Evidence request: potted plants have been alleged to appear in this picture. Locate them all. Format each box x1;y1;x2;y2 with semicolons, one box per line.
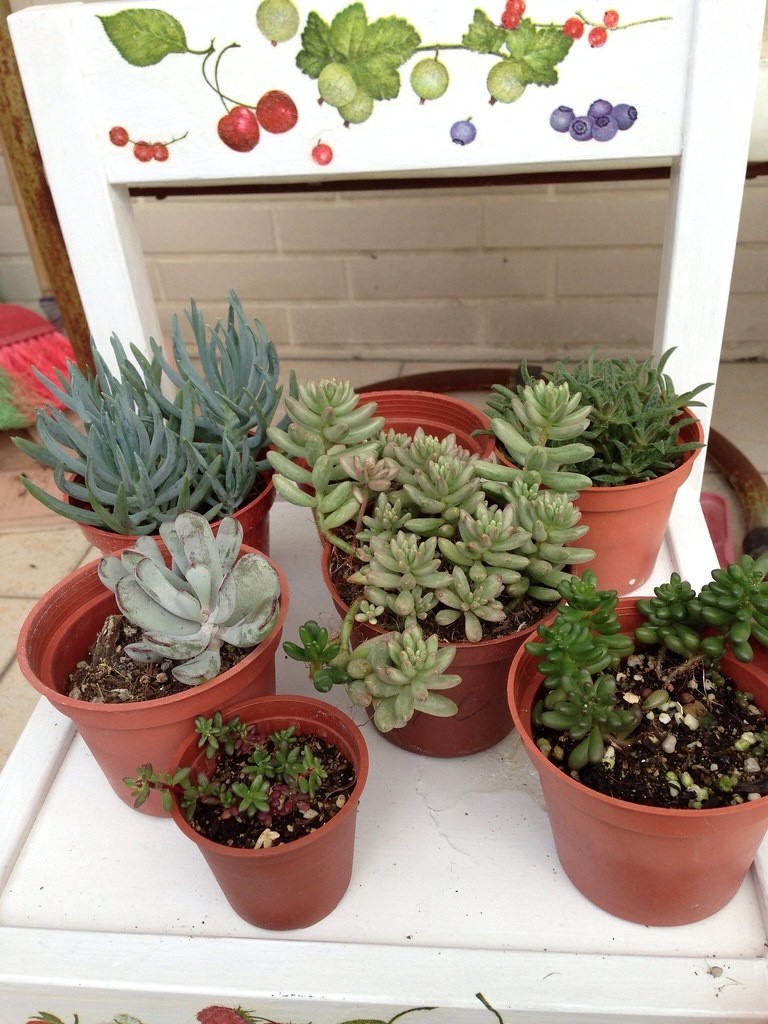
11;286;275;558
16;509;290;817
262;374;495;550
283;378;596;757
468;342;714;599
507;545;768;927
119;695;368;931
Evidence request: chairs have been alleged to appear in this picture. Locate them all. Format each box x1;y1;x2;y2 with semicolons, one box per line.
0;3;768;1024
